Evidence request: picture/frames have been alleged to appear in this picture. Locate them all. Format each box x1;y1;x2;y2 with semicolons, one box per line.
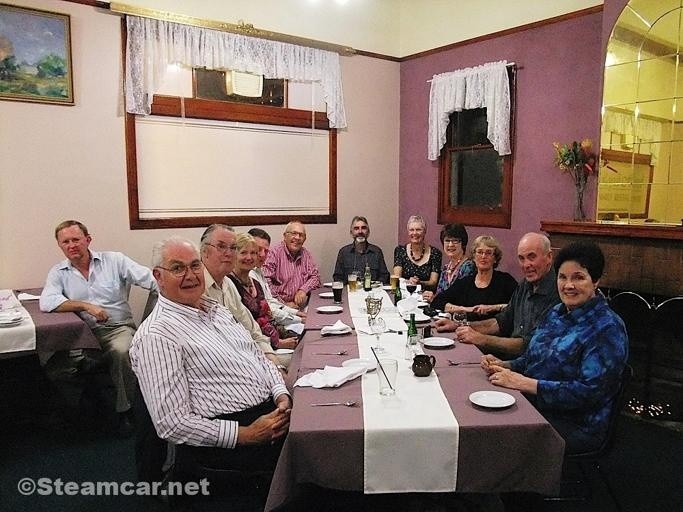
0;2;75;106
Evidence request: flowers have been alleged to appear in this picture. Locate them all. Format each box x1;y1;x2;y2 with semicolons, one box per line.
551;135;609;177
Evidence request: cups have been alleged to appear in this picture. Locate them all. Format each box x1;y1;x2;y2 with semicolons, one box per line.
332;271;467;397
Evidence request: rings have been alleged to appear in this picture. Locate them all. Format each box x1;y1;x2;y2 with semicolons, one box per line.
496;376;497;379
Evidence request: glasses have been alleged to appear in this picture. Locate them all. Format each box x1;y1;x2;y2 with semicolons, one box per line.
475;250;493;256
156;262;202;277
205;243;238;253
287;232;306;239
444;239;458;244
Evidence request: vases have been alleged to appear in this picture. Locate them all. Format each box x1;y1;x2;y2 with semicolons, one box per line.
572;175;588;222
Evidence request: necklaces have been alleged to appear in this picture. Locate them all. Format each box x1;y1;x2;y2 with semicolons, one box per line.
447;258;463;274
410;244;426;262
233;271;253;295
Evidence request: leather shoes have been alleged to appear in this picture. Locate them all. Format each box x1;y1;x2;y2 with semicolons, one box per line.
116;409;135;440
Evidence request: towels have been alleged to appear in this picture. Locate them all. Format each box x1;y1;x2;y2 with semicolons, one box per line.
396;295;423;316
291;362;377;389
320;319;352;339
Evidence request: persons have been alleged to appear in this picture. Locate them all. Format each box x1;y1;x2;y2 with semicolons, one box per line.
198;223;292;390
332;216;391;285
126;236;292;469
38;220;162;439
422;221;476;304
430;234;519;321
481;240;630;512
394;214;442;293
262;220;322;312
435;232;563;359
246;228;307;334
227;233;302;349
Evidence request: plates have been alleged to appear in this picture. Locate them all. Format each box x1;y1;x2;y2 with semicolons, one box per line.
317;282;516;408
0;310;24;327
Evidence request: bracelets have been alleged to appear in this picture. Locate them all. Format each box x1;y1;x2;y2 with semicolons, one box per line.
500;304;502;312
277;365;286;369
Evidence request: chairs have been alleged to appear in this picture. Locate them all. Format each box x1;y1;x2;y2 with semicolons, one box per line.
121;357;253;512
569;365;636;512
126;283;158;328
